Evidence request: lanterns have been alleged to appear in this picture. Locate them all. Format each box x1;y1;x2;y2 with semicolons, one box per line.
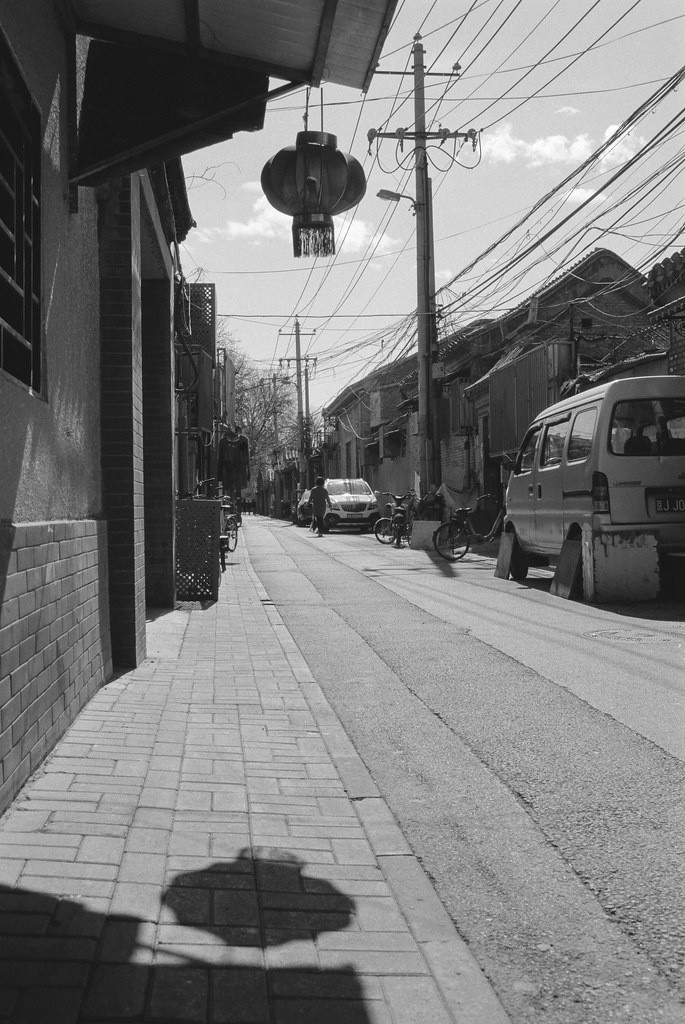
259;131;365;257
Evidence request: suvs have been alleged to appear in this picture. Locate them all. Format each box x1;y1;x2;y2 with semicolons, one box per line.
320;478;381;529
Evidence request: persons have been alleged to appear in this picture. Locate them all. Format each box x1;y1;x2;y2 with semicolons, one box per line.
240;498;256;515
306;477;332;536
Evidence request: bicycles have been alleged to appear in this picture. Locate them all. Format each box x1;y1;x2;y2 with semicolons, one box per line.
431;494;507;562
189;477;239;552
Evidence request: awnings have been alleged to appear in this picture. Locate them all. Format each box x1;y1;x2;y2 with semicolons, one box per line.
52;1;399;95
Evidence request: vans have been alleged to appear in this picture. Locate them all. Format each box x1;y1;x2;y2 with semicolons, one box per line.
503;375;685;582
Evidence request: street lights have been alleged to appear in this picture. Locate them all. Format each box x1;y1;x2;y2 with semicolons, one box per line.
376;186;428;497
281;380;306;494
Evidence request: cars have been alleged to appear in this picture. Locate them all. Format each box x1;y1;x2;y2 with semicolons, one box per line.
373;491;444;548
296;489;316;527
